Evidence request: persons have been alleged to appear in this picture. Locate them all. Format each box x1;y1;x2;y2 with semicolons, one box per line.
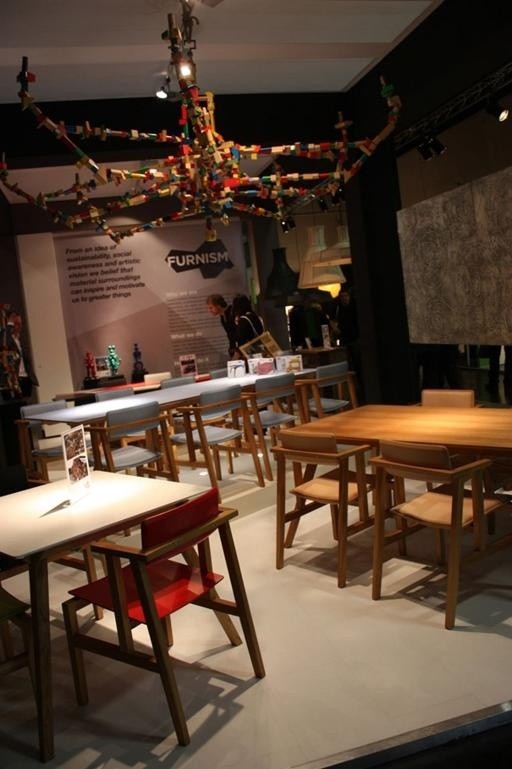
6;313;41;405
206;288;354;436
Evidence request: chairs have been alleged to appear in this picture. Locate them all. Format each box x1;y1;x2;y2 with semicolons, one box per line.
293;360;359;421
209;365;266;431
159;375;225;433
410;388;482;412
61;485;267;746
224;372;312;479
169;384;269;503
87;406;180;484
23;400;84;476
265;427;397;598
92;373;129;386
142;370;173;383
367;435;505;636
90;383;183;463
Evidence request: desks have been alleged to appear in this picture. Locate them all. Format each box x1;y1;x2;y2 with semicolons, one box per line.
21;361;351;478
277;402;510;563
42;361;242;395
0;465;208;750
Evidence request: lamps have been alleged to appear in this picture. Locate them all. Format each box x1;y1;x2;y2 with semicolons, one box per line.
2;2;409;261
